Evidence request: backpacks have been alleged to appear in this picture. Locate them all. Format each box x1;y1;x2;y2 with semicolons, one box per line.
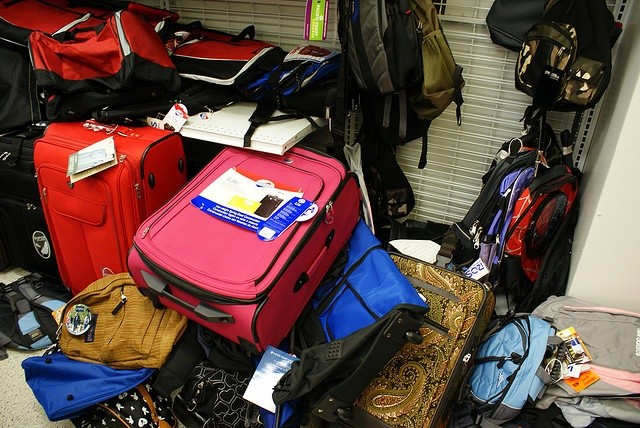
0;268;73;360
450;123;586;313
461;295;640;426
373;218;450;256
487;1;623;125
338;0;465;148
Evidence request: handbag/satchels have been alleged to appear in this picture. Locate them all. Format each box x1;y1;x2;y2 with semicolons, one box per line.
327;124;414;226
0;0;339;126
266;214;428;428
22;271;261;426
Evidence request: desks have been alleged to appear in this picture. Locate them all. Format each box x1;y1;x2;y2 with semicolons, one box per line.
138;99;330;169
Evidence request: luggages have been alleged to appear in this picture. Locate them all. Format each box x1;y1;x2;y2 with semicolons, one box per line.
33;120;186;298
0;125;49;269
124;143;363;364
338;249;495;427
